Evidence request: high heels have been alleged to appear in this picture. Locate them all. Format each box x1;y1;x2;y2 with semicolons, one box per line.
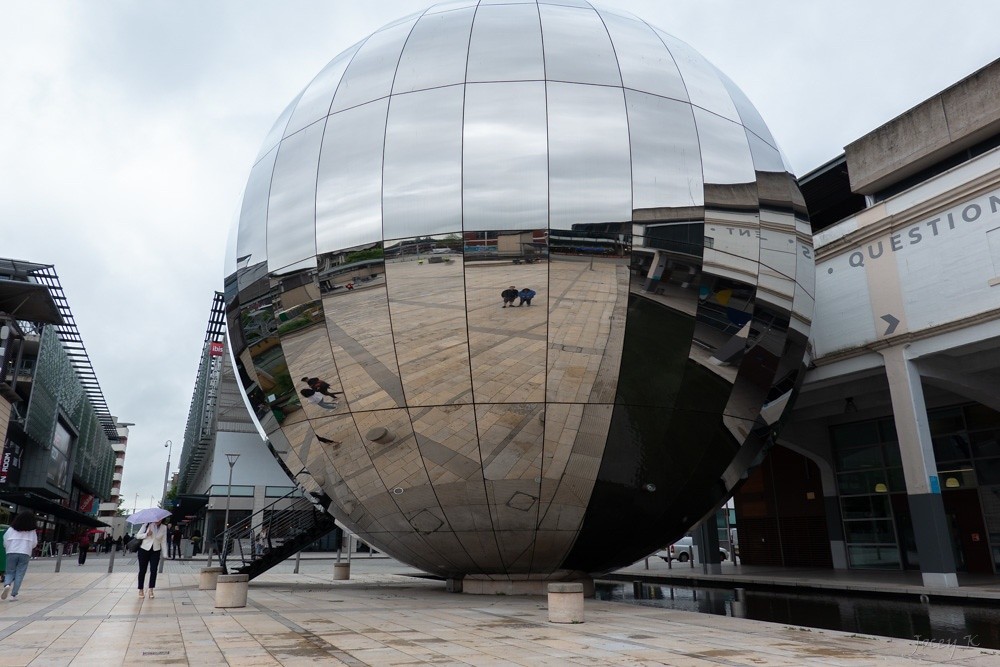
147;588;155;598
139;590;145;599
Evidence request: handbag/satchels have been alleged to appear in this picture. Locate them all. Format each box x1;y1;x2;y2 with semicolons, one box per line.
127;538;143;552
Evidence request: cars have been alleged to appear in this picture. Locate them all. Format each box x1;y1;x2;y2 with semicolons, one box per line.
656;537;730;563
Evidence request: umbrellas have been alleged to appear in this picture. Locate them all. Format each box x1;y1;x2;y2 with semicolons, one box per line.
89;529;104;533
126;508;172;525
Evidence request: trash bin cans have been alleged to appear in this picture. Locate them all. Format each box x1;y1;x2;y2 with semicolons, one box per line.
0;529;6;582
184;541;194;558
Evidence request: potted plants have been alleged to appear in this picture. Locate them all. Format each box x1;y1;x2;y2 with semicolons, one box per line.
192;536;200;556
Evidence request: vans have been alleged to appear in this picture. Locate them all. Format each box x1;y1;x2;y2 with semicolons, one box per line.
729;527;740;556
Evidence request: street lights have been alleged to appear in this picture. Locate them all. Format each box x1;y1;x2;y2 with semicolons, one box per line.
220;453;241;567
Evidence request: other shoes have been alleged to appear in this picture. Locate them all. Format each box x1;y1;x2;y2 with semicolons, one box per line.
517;304;523;308
526;305;531;308
1;586;10;600
331;397;341;400
502;305;515;308
335;401;339;409
333;440;342;446
10;595;19;602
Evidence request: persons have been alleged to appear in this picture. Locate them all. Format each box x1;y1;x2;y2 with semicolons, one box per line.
2;511;38;601
501;285;518;309
300;389;339;412
316;434;342;446
257;527;267;559
77;523;201;567
136;518;167;599
518;288;536;307
301;377;343;401
